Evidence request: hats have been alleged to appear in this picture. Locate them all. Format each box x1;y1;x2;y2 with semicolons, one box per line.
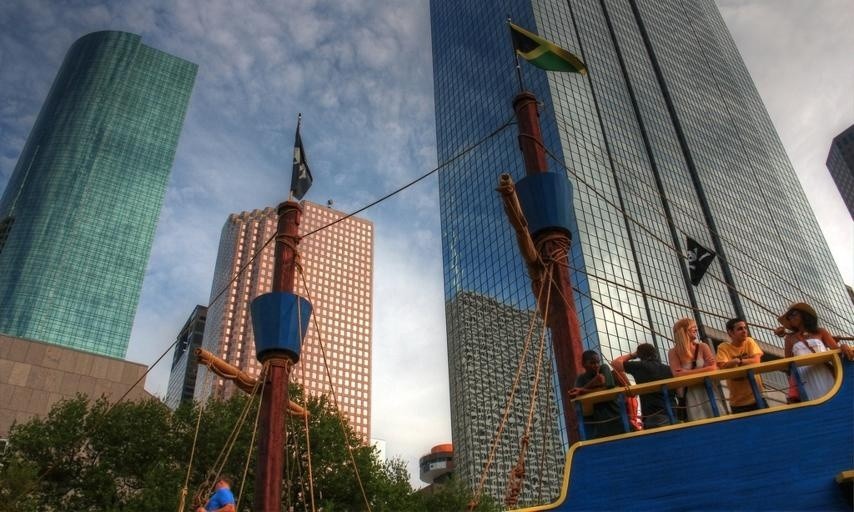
777;303;817;331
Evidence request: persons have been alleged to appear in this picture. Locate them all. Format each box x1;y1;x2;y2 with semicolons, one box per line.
567;303;854;438
195;474;237;512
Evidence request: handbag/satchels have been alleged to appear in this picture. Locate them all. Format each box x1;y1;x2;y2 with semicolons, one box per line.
677;398;688;420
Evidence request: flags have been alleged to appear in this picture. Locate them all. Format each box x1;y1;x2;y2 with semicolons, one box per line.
509;23;589;78
687;235;717;286
290;121;314;201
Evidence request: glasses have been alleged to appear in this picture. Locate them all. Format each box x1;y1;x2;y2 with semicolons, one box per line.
787;310;800;320
